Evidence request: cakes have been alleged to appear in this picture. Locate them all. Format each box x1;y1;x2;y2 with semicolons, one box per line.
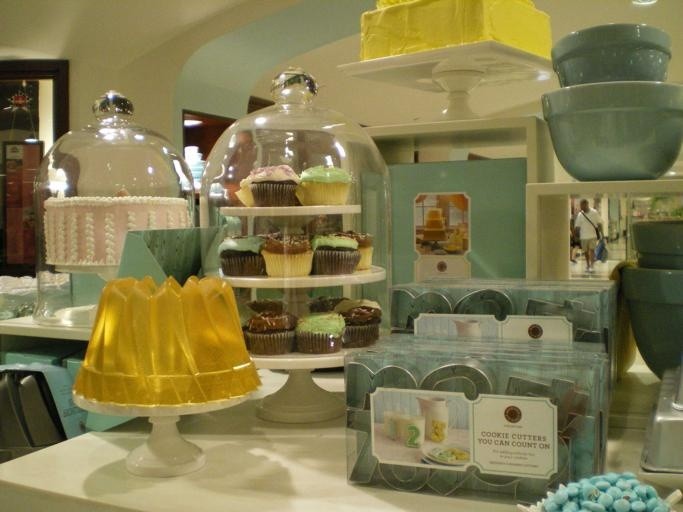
357;0;554;64
72;275;264;406
240;295;384;357
217;216;376;279
422;207;449;241
41;194;190;268
234;164;353;207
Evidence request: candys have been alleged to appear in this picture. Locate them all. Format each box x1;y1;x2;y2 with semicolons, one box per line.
541;470;671;512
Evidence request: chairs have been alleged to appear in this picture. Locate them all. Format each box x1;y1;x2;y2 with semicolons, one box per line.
0;368;69;461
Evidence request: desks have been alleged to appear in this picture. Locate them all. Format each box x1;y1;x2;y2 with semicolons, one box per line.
0;302;143;362
0;415;541;512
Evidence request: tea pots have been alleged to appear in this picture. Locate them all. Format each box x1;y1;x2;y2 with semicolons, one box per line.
416;396;460;444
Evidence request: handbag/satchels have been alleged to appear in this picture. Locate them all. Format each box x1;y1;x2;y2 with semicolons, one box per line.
595;225;600;239
593;241;608;262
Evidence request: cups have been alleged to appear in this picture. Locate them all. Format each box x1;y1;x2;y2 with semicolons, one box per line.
383;410;425;448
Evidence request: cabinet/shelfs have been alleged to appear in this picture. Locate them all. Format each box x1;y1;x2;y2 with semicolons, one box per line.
337;41;683;284
218;204;387;420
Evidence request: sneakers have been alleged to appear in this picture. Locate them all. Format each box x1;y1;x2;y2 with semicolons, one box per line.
585;265;595;273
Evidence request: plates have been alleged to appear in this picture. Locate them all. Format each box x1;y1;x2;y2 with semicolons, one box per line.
419;446;470;467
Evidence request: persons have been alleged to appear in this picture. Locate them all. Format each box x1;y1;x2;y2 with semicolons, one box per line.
570;207;581;264
575;199;604;273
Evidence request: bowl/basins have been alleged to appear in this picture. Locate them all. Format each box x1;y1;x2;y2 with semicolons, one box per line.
629;221;683;268
551;23;672;87
621;267;683;382
541;80;682;180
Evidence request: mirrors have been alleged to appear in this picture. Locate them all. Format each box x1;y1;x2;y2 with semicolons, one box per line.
0;60;69;280
